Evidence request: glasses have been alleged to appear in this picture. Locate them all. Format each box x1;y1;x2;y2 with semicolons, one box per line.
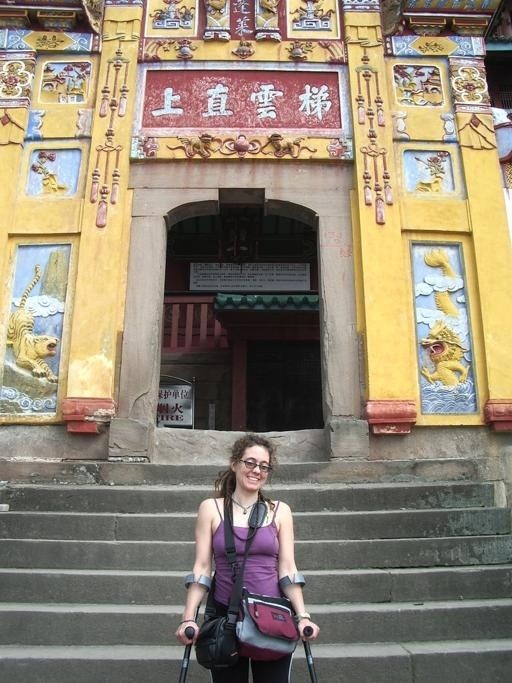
240;458;273;472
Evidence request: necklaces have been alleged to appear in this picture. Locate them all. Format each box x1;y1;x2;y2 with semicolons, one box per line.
232;496;258;516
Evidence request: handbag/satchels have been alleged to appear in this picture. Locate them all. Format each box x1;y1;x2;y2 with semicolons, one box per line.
195;587;300;668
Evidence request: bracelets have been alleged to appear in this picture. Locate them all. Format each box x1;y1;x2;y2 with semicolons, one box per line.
293;611;312;622
178;619;196;624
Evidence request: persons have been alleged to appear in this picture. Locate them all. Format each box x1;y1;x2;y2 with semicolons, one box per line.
175;434;319;683
